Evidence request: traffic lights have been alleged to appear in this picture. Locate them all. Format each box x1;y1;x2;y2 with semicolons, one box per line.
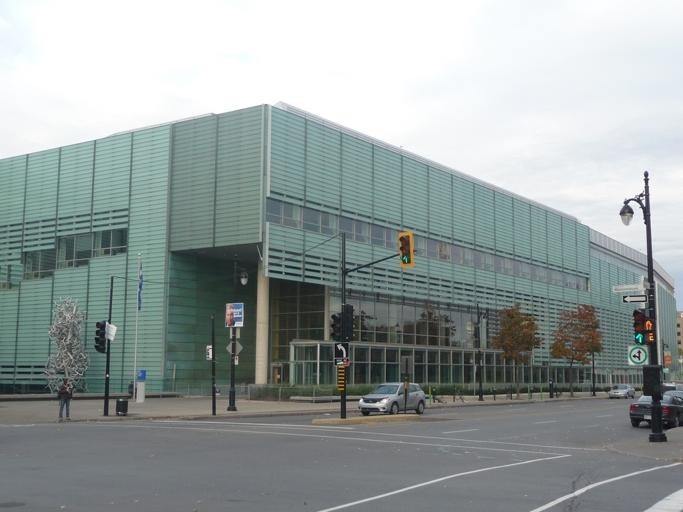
94;320;107;355
343;305;357;340
633;307;647;345
399;230;414;268
331;311;343;342
644;309;656;343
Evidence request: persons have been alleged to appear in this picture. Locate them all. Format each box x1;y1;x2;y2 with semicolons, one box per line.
226;304;235;326
58;378;72;420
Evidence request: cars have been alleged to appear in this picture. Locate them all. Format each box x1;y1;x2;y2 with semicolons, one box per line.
608;383;636;399
630;382;683;431
358;381;427;416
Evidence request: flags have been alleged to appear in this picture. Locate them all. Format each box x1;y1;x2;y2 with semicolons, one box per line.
663;350;672;368
137;257;145;310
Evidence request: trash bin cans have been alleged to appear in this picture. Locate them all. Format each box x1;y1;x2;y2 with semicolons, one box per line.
116;399;128;416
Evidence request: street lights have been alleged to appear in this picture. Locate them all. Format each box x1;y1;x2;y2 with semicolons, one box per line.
476;301;490;402
620;168;669;443
